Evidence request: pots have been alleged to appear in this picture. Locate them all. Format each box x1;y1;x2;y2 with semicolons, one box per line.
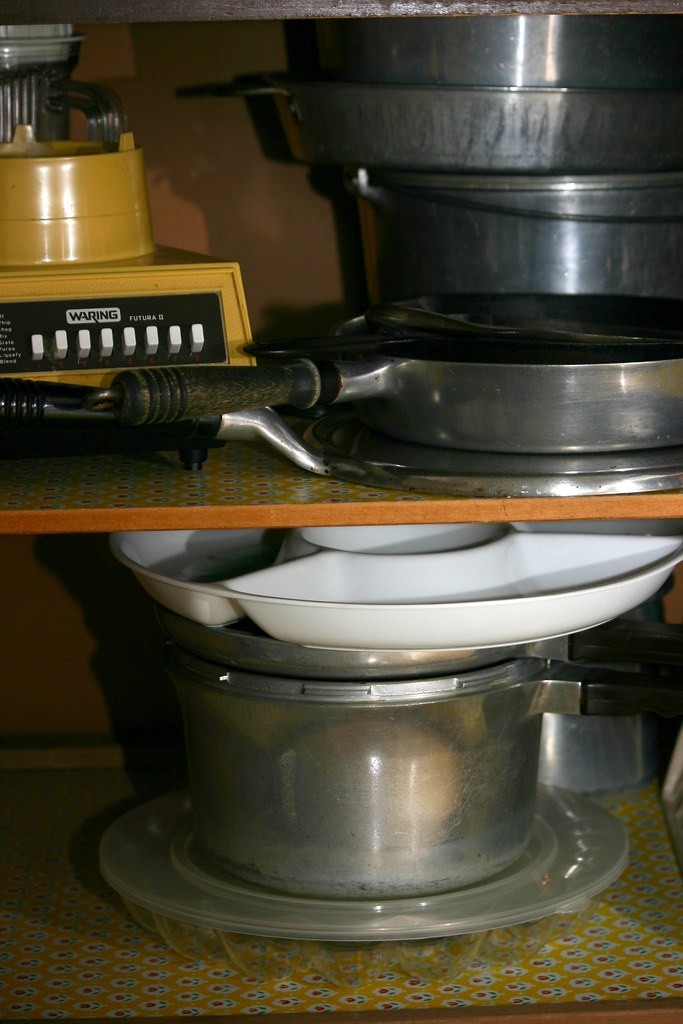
151;640;683;904
215;72;683;174
71;314;683;461
238;291;683;365
340;163;683;295
289;15;683;95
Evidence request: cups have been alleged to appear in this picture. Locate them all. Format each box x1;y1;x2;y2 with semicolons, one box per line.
1;65;128;144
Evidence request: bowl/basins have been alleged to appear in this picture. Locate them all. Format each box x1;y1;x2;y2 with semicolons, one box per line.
1;32;84;69
96;783;635;992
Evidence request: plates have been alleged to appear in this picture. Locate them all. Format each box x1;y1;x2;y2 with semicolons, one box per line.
111;516;683;657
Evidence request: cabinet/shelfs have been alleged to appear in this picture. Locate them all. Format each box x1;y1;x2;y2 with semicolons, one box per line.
0;2;683;1023
